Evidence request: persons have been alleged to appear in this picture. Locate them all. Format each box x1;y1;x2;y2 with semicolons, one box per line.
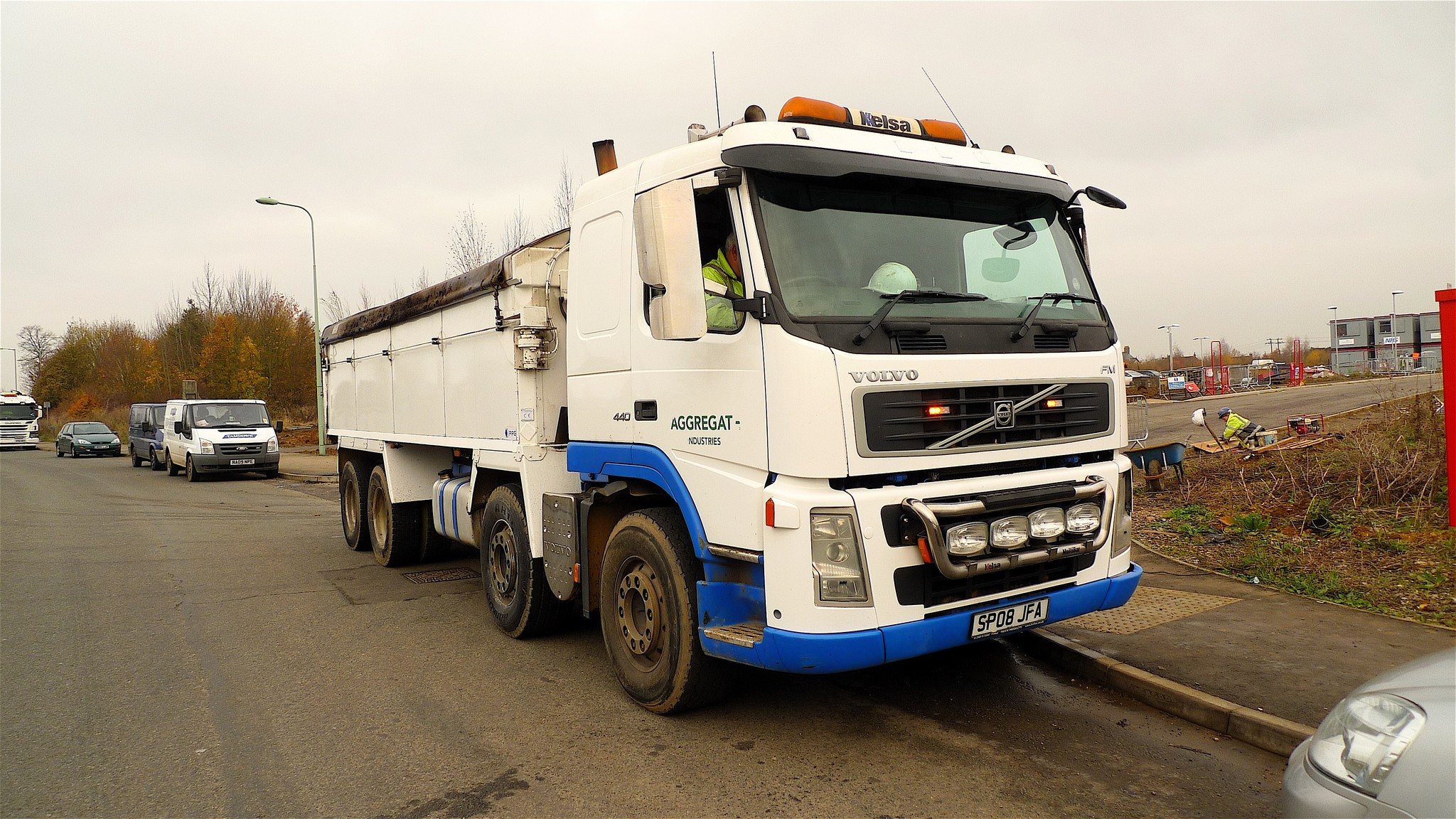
701;229;744;332
1399;355;1406;370
1216;407;1266;447
1385;360;1390;370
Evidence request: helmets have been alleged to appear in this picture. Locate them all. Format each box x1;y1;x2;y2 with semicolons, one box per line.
1217;407;1232;419
860;262;917;295
1191;408;1204;427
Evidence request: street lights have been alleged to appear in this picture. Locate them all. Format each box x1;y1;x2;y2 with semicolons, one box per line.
1391;289;1404;372
256;195;326;457
1327;304;1342;372
1191;336;1210;368
1157;324;1180;371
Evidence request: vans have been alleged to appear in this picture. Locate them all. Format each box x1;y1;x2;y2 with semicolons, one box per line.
127;403;174;470
162;398;284;482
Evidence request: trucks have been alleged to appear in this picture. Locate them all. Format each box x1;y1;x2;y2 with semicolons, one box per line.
0;389;43;449
316;95;1145;713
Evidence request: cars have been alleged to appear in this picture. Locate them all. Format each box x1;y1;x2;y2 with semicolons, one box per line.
1135;370;1162;377
1124;370;1150;386
54;419;122;458
